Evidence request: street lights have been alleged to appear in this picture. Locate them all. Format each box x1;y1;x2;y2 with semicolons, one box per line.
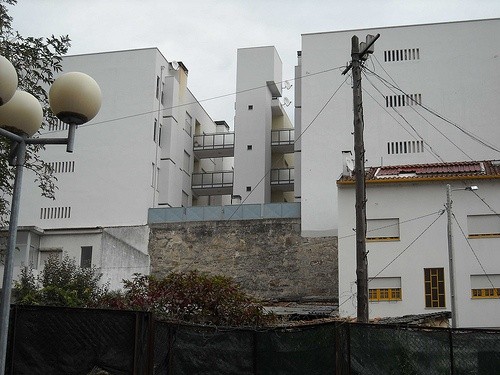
0;55;102;375
447;184;479;328
